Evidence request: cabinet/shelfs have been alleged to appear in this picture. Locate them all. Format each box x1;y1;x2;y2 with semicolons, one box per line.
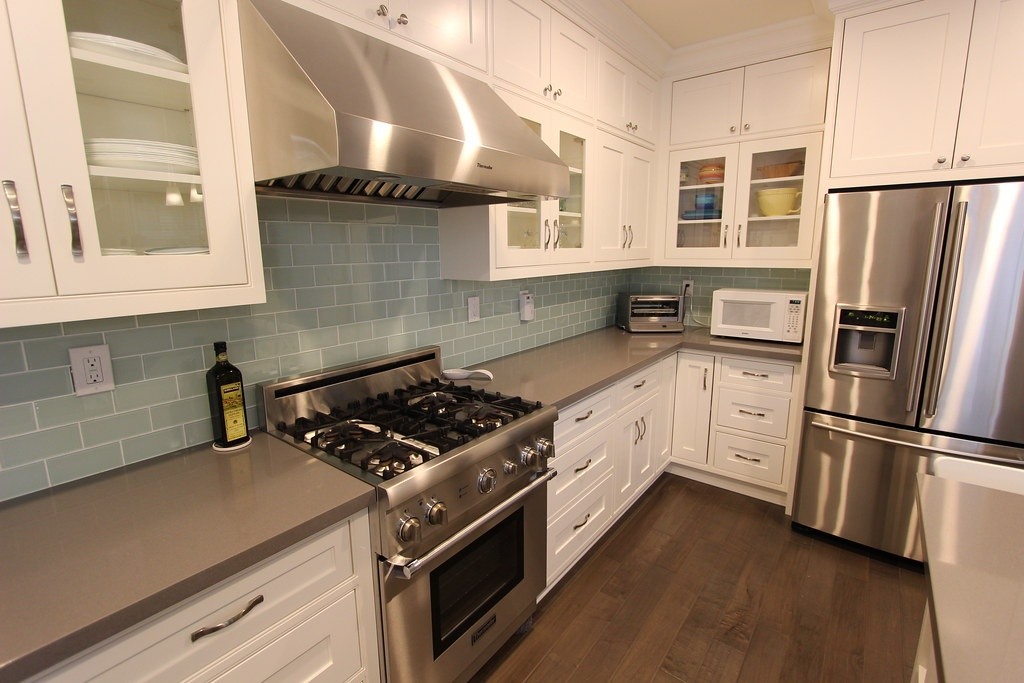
281;0;659;282
23;507;381;683
672;353;714;464
0;0;266;328
547;354;678;585
665;48;831;259
830;0;1023;178
713;357;794;485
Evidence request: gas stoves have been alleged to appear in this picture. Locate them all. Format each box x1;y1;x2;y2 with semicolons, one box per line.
254;345;559;511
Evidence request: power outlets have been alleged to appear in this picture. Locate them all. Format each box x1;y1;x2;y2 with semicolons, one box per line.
69;344;115;397
682;280;694;296
468;296;480;322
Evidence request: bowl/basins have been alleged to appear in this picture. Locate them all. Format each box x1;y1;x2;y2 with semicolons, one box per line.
755;162;801;178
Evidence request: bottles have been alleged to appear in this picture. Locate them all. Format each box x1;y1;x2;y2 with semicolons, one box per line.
205;341;250;448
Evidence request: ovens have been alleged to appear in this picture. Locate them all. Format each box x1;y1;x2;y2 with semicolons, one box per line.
371;467;548;683
616;292;685;332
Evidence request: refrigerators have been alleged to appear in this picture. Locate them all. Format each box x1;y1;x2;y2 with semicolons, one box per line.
789;180;1024;563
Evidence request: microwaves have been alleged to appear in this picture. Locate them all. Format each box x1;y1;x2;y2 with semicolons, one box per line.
709;288;808;344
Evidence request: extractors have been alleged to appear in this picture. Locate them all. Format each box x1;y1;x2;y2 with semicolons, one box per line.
235;0;570;209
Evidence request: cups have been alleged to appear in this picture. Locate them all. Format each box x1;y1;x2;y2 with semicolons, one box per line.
695;194;716;210
755;188;802;216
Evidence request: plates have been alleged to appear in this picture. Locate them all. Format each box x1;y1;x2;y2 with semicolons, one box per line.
682;209;722;220
84;138;200;176
68;31;188;73
100;246;210;256
